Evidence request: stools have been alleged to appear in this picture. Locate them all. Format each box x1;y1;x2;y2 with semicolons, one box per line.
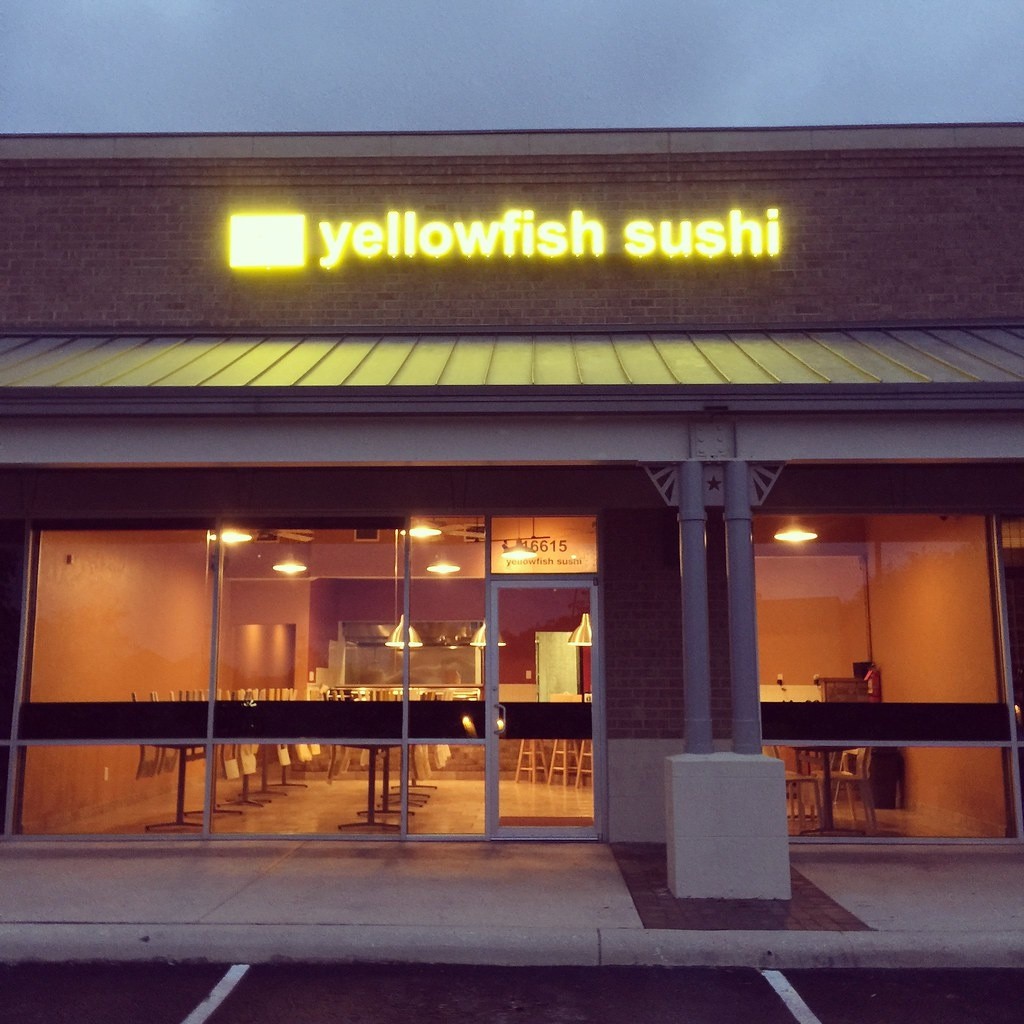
514;739;593;788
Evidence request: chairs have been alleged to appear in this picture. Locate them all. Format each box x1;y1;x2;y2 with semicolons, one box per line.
785;746;877;831
130;687;451;784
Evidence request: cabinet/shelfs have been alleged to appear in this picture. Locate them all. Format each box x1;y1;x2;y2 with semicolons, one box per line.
818;678;869;701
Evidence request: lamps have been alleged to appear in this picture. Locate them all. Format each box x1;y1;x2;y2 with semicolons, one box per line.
501;539;536;559
427;559;461;573
567;612;592;646
774;517;817;540
400;520;442;538
384;614;424;648
469;618;506;647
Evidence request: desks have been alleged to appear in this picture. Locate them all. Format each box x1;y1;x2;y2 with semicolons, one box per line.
146;745;436;832
792;747;865;835
